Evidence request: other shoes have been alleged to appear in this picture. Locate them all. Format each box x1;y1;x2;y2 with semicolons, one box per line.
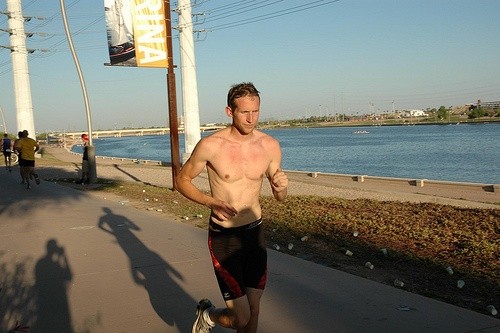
35;177;40;184
29;176;33;180
27;184;31;189
20;181;25;184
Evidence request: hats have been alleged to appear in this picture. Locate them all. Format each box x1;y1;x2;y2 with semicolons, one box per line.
81;134;89;140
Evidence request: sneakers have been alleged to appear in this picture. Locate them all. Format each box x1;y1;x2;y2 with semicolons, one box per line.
192;299;216;333
